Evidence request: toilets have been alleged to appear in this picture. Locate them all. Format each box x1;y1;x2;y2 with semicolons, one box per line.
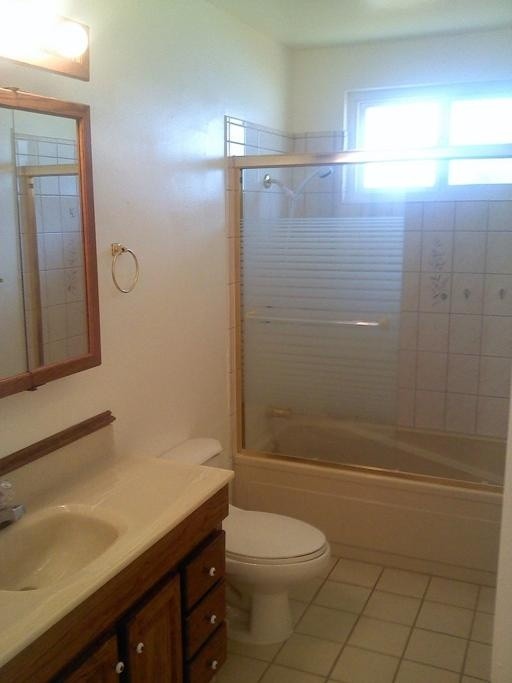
158;437;331;646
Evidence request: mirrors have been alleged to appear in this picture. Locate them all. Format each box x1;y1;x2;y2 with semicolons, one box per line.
0;86;101;399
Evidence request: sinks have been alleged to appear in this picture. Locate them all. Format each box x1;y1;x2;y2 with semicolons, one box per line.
0;505;127;591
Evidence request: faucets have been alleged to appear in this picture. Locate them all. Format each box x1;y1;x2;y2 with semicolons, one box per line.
0;480;25;530
269;405;292;420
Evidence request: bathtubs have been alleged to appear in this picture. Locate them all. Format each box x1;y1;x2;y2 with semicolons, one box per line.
234;415;507;505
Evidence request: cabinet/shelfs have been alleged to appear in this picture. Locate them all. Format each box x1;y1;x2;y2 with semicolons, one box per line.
0;485;230;683
